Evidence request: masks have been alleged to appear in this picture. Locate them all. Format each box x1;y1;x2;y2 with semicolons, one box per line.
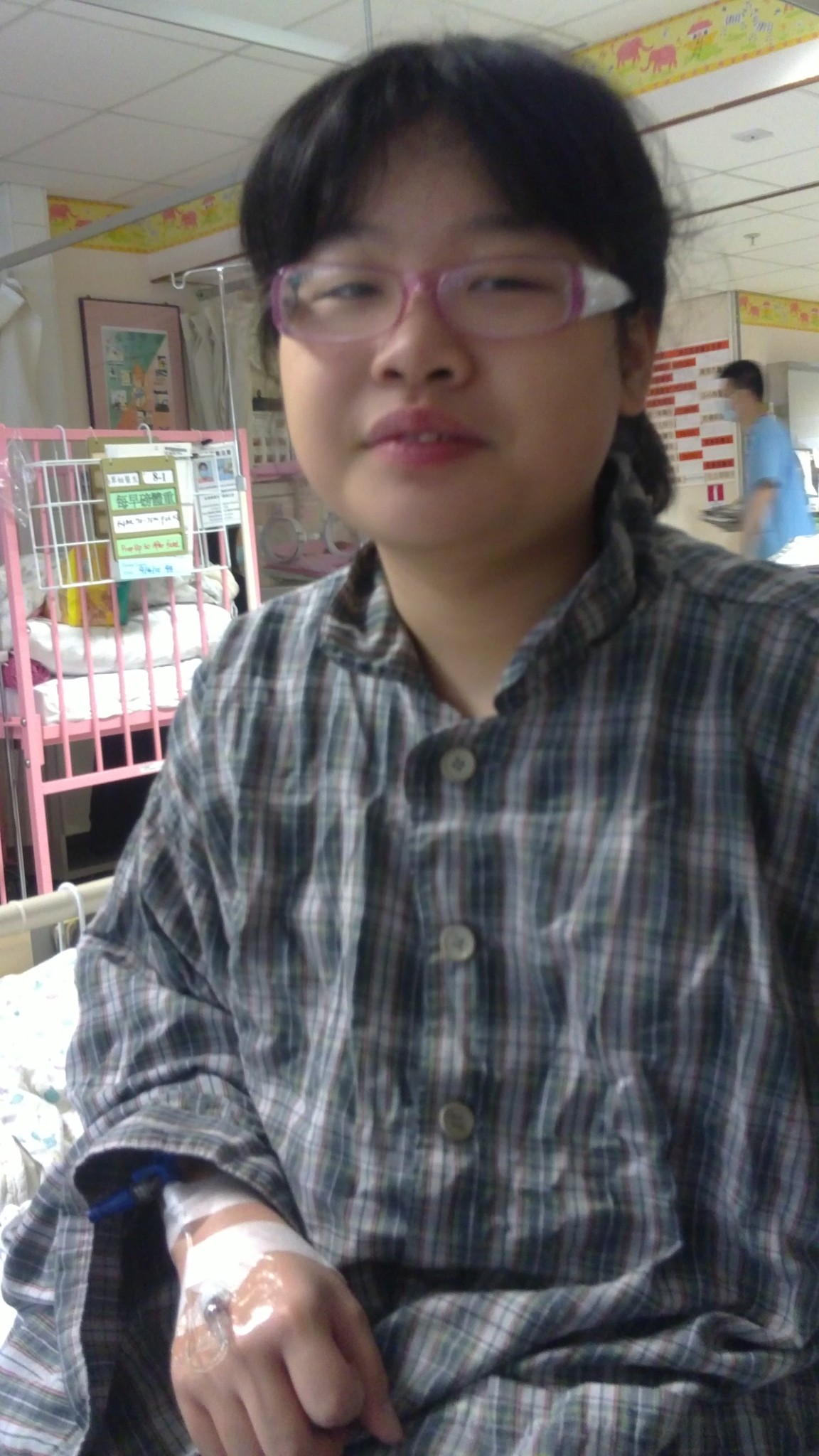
719;393;748;421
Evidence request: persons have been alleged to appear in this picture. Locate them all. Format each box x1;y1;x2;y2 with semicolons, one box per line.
717;360;817;564
198;463;214;482
0;36;819;1456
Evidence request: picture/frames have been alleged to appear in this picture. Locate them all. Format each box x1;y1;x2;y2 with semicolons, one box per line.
77;294;191;430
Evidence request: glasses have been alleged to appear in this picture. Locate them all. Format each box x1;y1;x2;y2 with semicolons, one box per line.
270;260;634;343
